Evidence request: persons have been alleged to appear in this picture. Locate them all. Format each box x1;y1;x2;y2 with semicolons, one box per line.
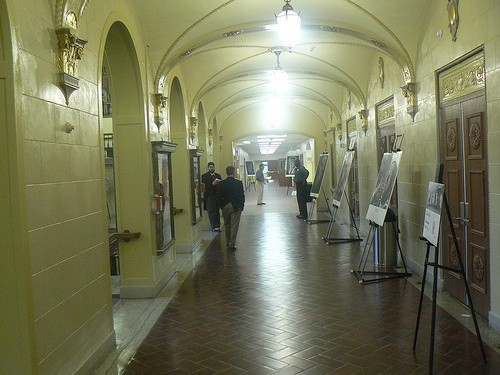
289;156;309;219
210;166;245;249
255;164;266;205
201;162;222;232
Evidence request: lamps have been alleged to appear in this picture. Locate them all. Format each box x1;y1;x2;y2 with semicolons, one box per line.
274;0;303;48
